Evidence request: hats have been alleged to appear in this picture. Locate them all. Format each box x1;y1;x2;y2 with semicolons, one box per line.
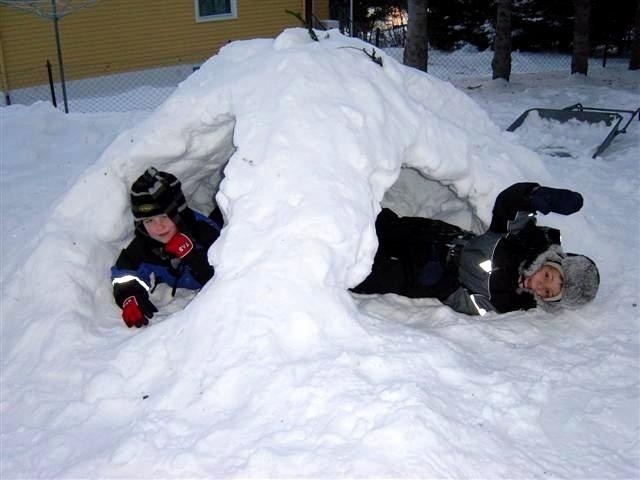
129;166;187;221
524;245;600;313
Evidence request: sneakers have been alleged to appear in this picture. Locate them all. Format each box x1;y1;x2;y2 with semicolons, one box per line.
349;270;391;299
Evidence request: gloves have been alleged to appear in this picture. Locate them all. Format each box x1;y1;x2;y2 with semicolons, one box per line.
165;232;214;284
530;185;583;215
418;261;450;297
113;280;154;328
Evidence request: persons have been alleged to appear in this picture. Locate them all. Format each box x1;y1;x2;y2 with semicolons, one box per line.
108;165;228;331
345;181;601;317
